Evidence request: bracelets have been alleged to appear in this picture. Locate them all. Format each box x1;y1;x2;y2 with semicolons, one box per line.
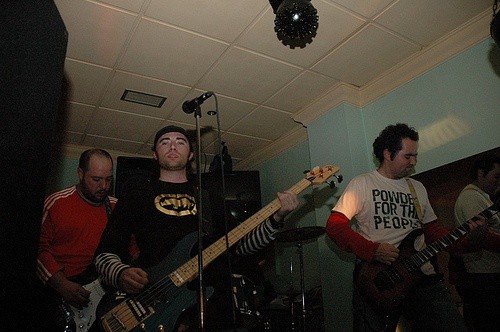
269;214;284;230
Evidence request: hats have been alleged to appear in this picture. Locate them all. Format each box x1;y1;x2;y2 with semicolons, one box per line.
154;125;192;149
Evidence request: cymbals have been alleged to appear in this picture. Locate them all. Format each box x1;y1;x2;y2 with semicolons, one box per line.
275;226;326;243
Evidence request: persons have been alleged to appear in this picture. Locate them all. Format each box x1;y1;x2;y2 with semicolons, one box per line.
325;123;490;332
454;153;500;332
36;148;118;332
94;124;301;332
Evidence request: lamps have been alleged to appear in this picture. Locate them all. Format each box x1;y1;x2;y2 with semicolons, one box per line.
268;0;319;49
489;0;500;46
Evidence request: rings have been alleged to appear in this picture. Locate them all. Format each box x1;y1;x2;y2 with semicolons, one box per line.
80;285;82;290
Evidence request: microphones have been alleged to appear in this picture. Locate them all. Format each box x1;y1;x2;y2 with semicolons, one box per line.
182;92;213;114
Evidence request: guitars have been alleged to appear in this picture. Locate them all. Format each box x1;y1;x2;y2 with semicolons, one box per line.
353;200;500;319
46;266;121;332
95;165;344;332
